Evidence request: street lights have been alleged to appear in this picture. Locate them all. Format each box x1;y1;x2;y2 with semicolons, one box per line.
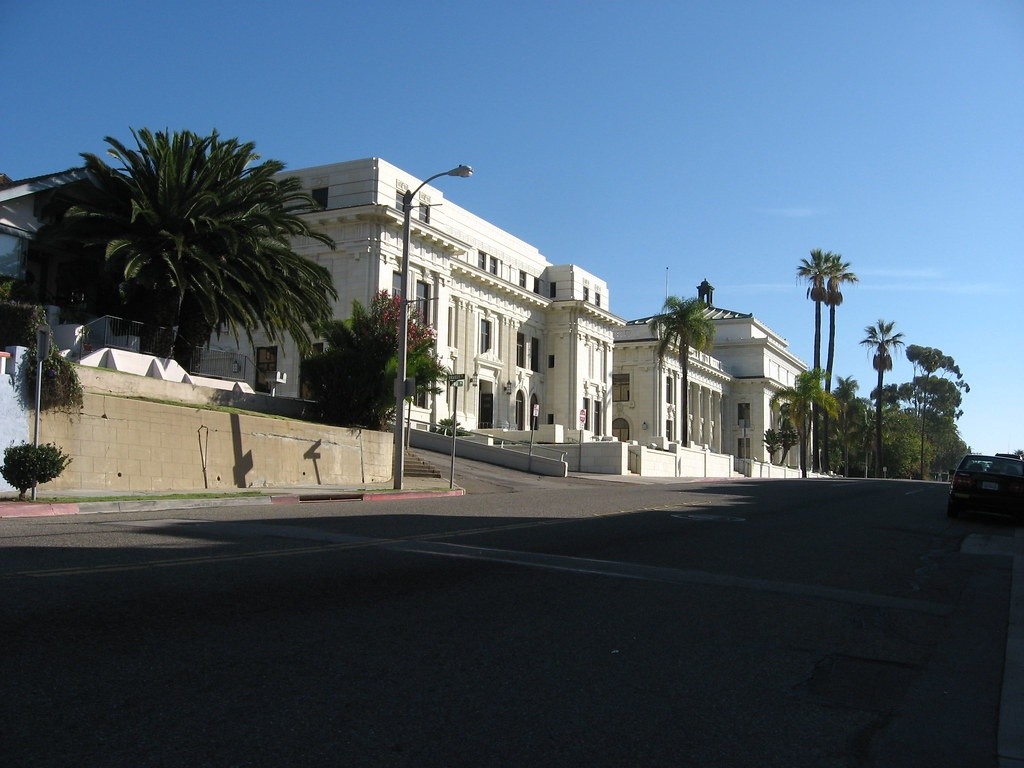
393;163;475;489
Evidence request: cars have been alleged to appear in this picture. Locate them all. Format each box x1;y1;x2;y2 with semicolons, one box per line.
989;452;1024;479
945;454;1024;522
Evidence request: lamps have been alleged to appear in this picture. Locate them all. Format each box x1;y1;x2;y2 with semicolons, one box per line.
470;371;478;386
504;380;512;395
231;360;241;373
